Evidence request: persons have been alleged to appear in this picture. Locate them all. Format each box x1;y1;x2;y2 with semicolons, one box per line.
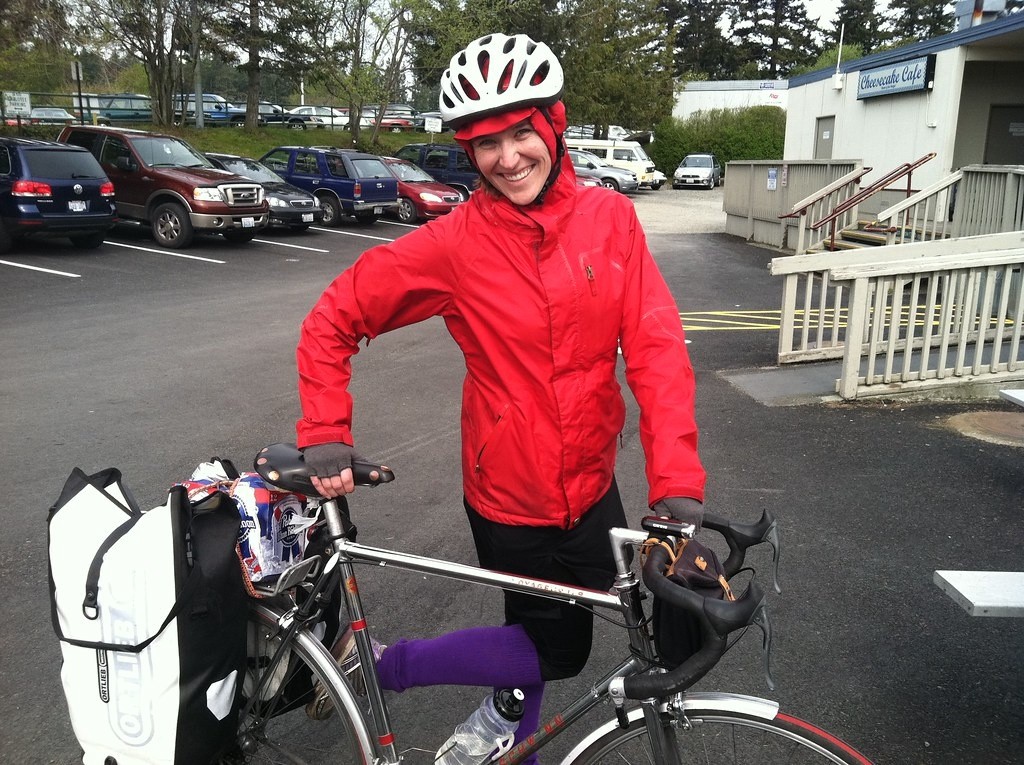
295;32;709;765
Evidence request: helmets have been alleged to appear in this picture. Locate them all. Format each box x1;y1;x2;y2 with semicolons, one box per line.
439;33;564;131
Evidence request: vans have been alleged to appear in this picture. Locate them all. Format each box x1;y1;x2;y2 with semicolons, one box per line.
651;170;668;190
565;138;656;187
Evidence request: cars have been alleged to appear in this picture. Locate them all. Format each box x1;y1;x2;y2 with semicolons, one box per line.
567;150;639;193
30;106;76;125
77;92;155;128
575;172;604;188
383;155;465;224
233;102;325;136
286;105;370;132
672;155;721;190
375;104;445;134
0;136;117;249
172;95;246;128
336;108;415;134
202;153;324;237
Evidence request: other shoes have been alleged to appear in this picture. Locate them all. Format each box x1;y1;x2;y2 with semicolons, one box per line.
306;624;387;720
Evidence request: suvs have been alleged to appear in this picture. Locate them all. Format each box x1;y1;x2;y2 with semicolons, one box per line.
257;144;403;227
55;124;270;249
392;143;483;202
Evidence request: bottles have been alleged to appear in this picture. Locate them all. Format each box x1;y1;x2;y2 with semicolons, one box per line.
435;688;525;765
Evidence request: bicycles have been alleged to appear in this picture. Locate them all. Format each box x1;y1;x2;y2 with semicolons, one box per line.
231;440;875;765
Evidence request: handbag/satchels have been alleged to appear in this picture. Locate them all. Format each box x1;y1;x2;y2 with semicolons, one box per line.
46;467;247;765
171;456;357;718
